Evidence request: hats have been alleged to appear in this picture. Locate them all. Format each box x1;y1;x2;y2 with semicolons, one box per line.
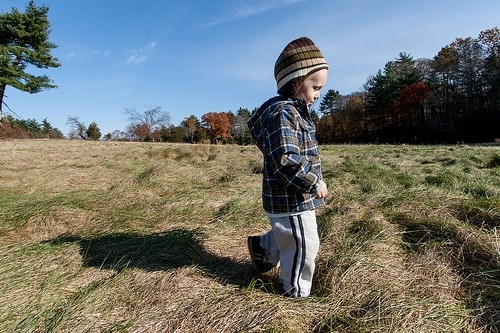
274;37;328;96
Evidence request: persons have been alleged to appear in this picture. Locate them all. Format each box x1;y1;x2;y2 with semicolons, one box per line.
248;37;328;299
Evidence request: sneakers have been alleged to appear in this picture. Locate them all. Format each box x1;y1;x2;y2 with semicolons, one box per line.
248;236;264;274
